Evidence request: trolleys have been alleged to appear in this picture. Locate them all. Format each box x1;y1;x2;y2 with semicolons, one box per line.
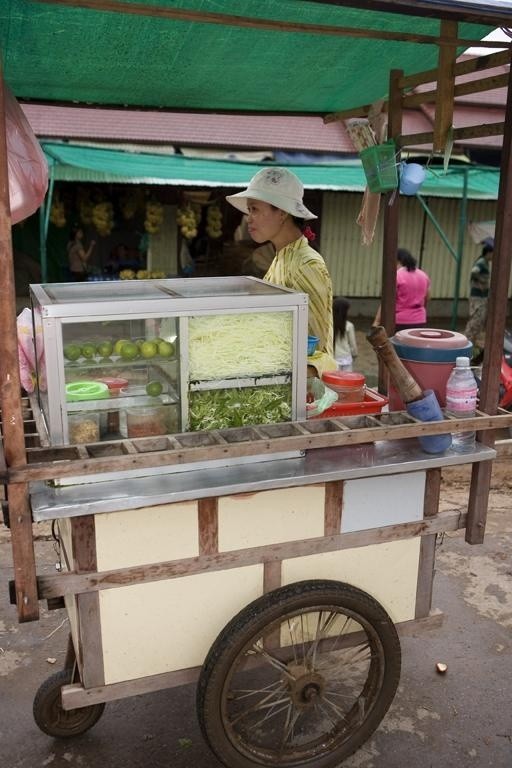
15;440;498;768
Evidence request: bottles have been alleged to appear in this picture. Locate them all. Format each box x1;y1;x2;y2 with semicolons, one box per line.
321;371;366;402
444;357;477;454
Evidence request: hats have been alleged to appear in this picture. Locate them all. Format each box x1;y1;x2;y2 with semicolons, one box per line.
224;166;319;221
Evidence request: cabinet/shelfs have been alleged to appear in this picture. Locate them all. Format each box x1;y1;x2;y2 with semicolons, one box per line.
29;275;310;486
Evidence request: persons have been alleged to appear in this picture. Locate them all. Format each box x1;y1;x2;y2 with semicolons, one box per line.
67;229;97;279
330;298;359;376
458;247;492;350
370;248;430;337
117;243;130;263
223;166;338;383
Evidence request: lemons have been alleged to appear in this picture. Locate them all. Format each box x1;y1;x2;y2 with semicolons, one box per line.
146;381;163;397
64;338;174;362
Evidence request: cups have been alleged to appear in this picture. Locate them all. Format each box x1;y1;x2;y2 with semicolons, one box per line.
406;390;452;453
400;161;426;196
